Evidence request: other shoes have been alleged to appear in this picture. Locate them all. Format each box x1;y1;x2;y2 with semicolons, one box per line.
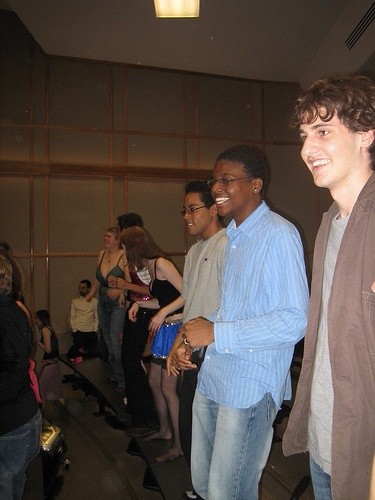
184;490;204;500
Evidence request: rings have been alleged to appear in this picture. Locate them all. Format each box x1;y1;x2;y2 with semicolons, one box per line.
183;338;189;346
181;331;185;339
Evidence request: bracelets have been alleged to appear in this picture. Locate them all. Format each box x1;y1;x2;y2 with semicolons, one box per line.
135;301;140;308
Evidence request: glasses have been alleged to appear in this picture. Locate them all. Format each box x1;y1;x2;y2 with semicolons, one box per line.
180;205;207;217
206;176;250;188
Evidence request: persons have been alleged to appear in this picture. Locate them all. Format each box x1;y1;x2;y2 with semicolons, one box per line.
176;147;309;500
282;77;375;500
0;179;230;500
